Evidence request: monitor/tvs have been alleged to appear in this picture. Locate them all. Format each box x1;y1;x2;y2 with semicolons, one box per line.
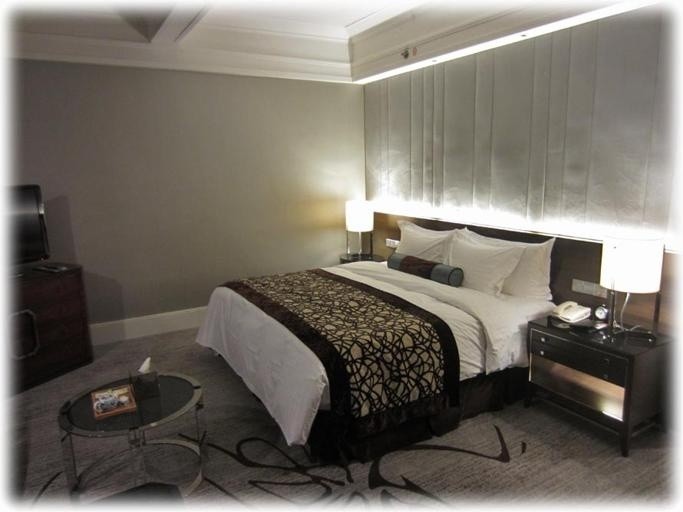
8;183;51;265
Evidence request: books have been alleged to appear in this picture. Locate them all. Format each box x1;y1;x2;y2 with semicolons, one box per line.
90;383;136;422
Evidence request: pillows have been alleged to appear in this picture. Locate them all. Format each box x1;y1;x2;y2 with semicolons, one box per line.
387;220;557;302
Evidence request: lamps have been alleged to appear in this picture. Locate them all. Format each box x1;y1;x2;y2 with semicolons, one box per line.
599;228;663;344
345;200;375;258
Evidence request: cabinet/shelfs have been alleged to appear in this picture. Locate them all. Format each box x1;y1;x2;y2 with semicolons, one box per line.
12;264;93;397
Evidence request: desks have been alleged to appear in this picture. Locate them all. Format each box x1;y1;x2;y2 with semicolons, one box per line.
57;369;208;501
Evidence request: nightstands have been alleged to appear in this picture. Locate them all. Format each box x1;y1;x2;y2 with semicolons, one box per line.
524;306;671;457
340;254;384;264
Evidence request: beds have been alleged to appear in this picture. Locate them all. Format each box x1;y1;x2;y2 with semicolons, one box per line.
215;212;603;468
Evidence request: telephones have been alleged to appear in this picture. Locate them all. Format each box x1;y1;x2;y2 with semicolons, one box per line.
549;301;592;324
